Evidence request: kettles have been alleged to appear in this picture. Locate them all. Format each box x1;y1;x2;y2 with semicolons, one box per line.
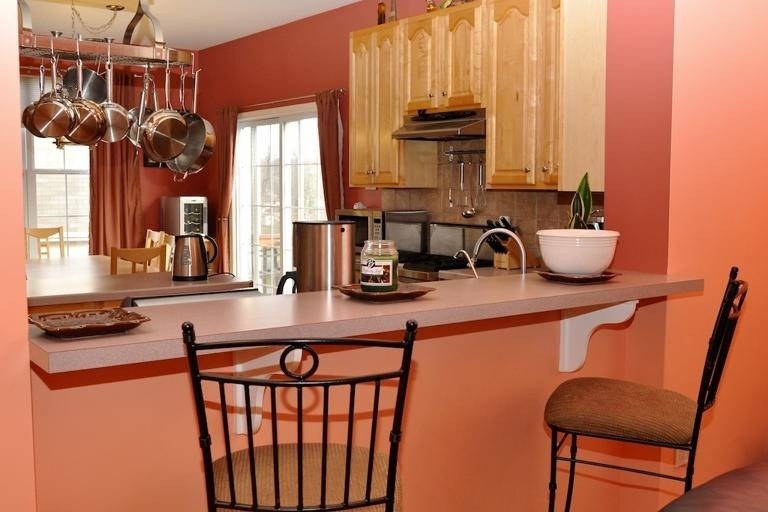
172;233;218;282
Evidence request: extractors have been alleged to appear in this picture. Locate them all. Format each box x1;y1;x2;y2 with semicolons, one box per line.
390;113;485;142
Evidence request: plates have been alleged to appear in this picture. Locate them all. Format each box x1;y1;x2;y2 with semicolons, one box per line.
29;307;152;335
536;269;623;284
334;280;437;301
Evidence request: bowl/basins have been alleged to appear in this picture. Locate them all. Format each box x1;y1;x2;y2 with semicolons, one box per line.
536;226;620;275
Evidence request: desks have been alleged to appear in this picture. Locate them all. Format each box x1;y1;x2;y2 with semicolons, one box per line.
26;254;160;279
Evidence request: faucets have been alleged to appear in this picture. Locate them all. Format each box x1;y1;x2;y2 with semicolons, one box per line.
466;228;527;274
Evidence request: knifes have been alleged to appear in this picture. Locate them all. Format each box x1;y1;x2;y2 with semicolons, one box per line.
481;217;515;251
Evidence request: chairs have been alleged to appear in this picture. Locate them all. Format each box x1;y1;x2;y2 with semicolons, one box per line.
182;316;418;512
145;229;175;272
111;245;166;275
24;226;64;260
545;266;750;511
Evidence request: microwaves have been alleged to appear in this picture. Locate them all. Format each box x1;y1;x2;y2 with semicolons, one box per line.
333;208;383;255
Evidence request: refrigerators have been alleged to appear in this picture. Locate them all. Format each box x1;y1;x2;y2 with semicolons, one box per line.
159;193;210;238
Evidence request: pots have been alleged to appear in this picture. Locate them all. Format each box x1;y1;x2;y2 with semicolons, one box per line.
23;54;217;183
